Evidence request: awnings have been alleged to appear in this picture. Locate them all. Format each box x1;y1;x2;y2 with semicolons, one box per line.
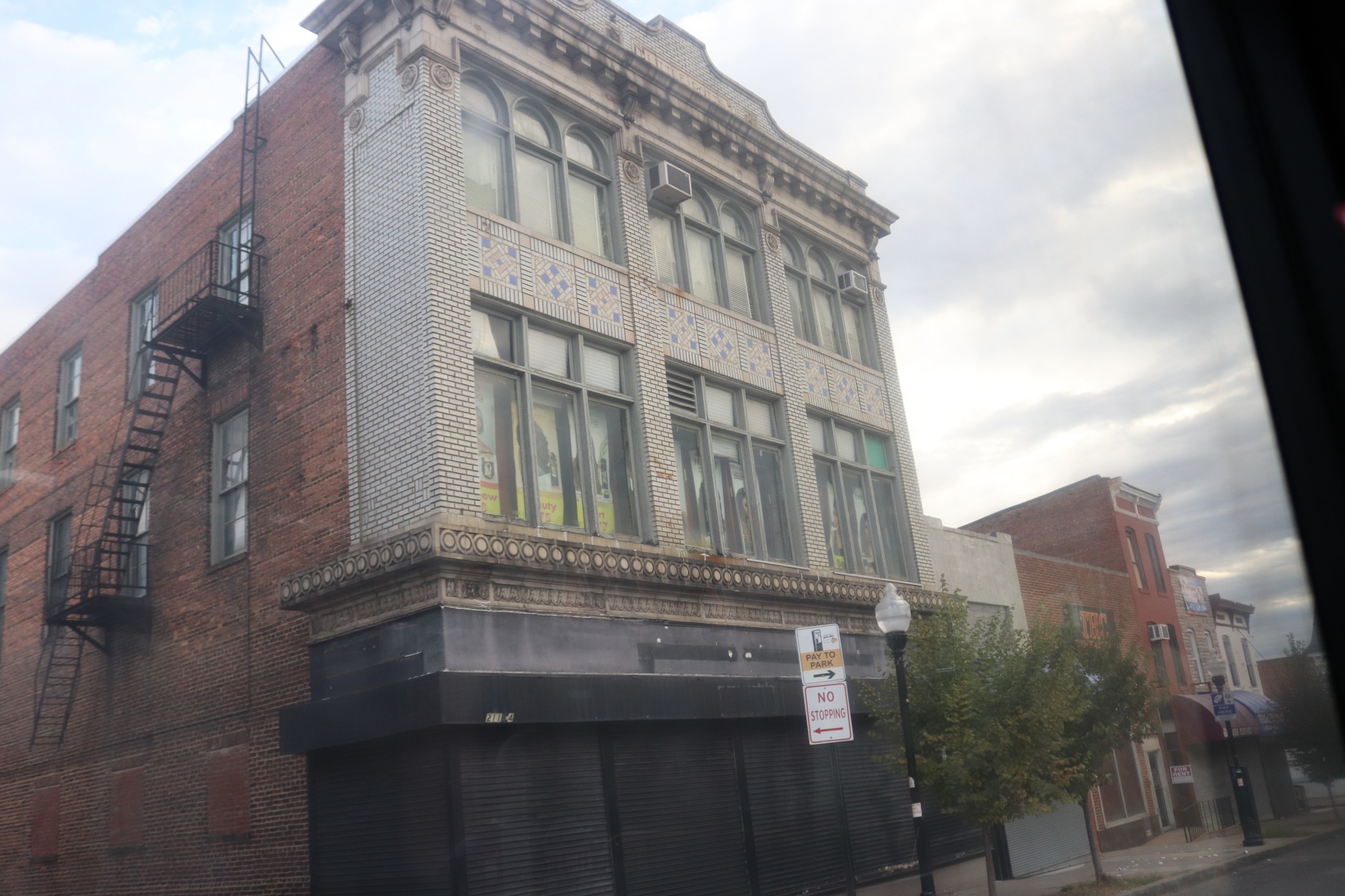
1172;690;1292;746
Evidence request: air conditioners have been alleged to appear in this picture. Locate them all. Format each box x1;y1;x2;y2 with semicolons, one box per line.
837;269;868;297
1149;624;1170;641
643;160;693;205
